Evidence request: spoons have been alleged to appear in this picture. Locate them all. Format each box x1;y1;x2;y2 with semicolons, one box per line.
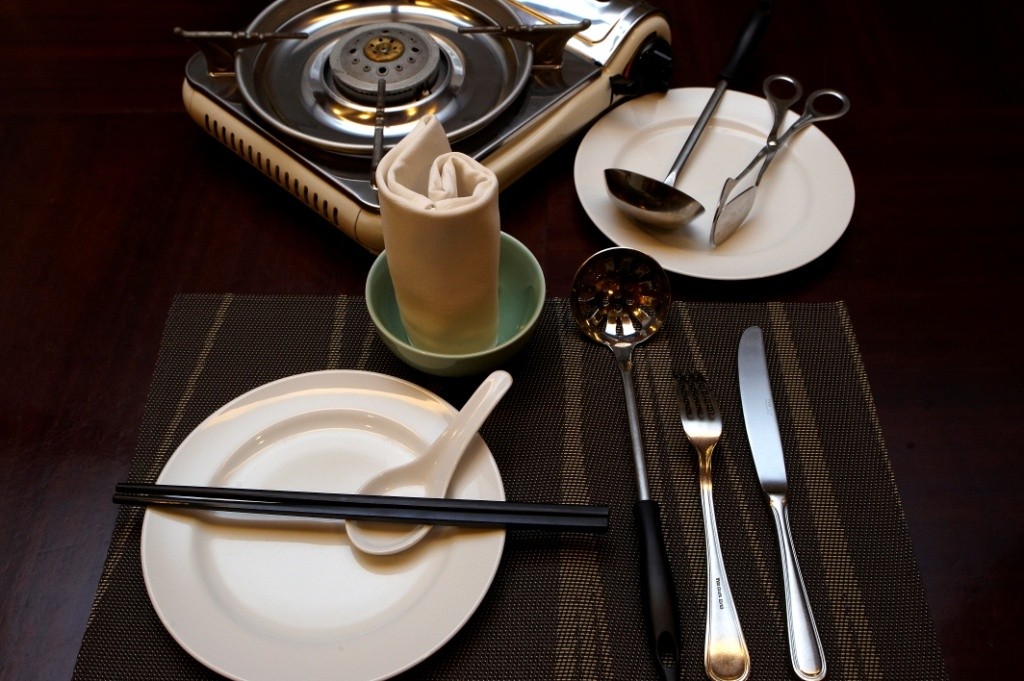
349;371;514;556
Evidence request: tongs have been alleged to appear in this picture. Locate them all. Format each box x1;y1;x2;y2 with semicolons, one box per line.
711;73;852;249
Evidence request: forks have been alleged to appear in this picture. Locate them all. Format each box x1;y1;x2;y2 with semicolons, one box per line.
670;366;751;681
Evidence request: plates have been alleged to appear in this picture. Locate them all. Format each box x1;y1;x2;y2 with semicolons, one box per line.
573;84;856;279
138;369;508;680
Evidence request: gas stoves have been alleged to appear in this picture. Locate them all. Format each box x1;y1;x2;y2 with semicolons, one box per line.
173;0;680;243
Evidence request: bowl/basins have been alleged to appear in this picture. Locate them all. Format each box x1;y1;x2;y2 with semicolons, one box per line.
362;229;545;378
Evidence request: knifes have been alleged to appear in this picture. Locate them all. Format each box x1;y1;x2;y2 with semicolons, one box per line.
735;325;827;680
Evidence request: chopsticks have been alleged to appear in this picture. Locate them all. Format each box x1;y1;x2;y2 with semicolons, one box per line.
108;479;610;540
